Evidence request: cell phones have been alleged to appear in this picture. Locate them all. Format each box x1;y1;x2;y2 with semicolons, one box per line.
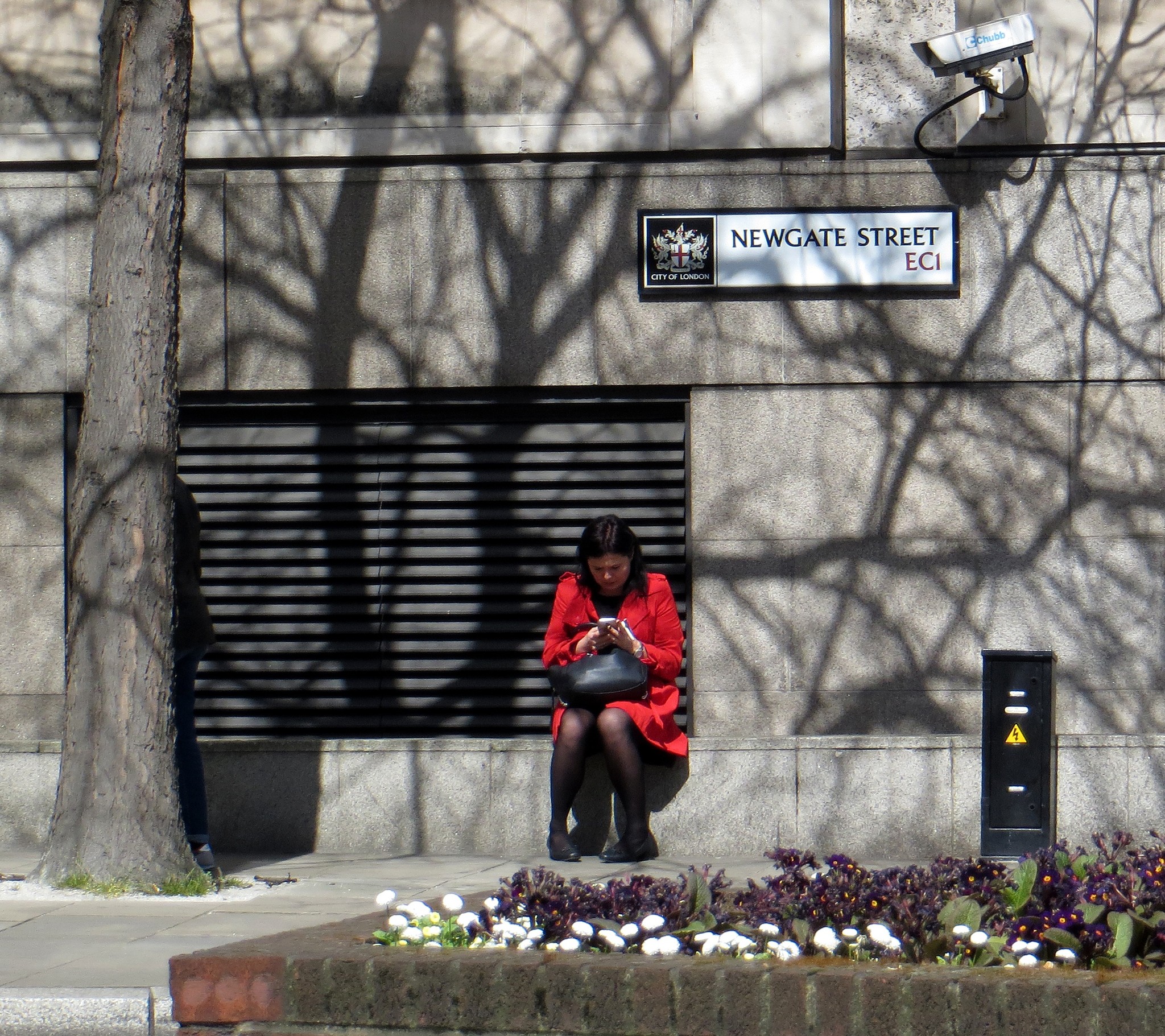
598;617;618;636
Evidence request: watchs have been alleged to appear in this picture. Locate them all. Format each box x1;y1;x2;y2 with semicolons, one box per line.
633;642;644;658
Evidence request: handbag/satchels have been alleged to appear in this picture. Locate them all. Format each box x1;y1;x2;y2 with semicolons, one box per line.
548;623;649;706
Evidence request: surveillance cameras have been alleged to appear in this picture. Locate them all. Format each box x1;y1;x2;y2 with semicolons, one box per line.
912;13;1035;80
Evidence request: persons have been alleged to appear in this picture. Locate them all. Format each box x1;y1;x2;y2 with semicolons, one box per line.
541;514;688;862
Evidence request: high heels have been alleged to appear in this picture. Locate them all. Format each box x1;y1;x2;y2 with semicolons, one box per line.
547;829;581;861
598;829;649;862
